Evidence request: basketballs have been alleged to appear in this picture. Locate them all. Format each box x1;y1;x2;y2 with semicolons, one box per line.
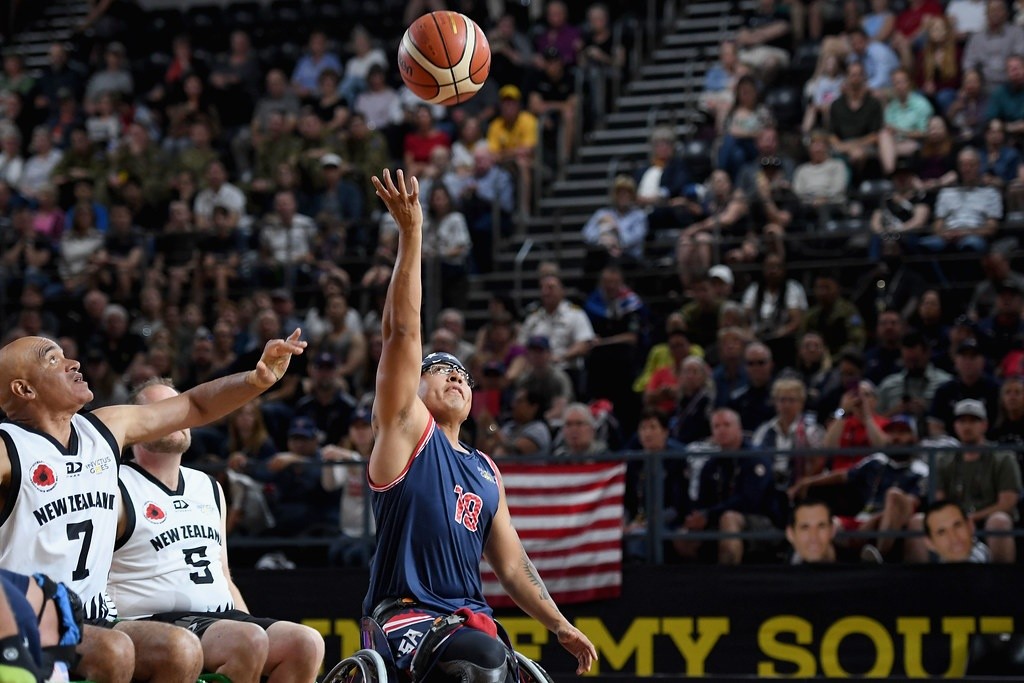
396;12;492;102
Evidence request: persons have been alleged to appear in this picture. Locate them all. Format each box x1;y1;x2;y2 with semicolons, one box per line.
922;499;994;564
0;326;308;683
787;498;843;565
106;377;325;683
361;168;599;683
0;0;1024;570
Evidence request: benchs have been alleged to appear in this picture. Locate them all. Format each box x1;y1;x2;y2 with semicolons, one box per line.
0;0;1024;561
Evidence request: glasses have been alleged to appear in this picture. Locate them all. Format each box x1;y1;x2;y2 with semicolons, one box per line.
746;357;767;368
423;365;475;387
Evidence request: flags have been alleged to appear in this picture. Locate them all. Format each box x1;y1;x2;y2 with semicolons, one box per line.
479;460;628;608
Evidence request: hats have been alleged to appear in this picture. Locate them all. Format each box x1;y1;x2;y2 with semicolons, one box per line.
312;351;338;369
108;41;124;56
708;264;734;284
501;84;521;100
527;336;551;350
954;337;981;354
421;352;466;373
882;414;918;437
350;409;372;424
287;417;316;437
954;399;987;420
320;152;343;167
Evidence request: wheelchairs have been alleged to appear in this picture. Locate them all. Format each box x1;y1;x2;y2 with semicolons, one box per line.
323;615;555;683
